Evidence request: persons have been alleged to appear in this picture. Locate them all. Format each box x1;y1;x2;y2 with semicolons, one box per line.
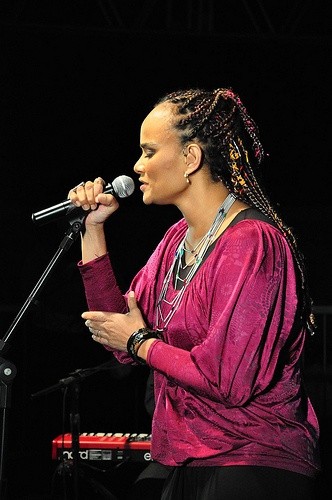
69;89;324;500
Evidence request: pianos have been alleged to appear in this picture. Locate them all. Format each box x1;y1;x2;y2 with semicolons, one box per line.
51;428;158;464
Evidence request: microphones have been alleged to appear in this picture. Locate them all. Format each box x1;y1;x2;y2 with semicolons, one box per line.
31;174;135;222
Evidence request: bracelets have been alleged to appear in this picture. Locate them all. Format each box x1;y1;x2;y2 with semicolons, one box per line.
126;327;160;359
